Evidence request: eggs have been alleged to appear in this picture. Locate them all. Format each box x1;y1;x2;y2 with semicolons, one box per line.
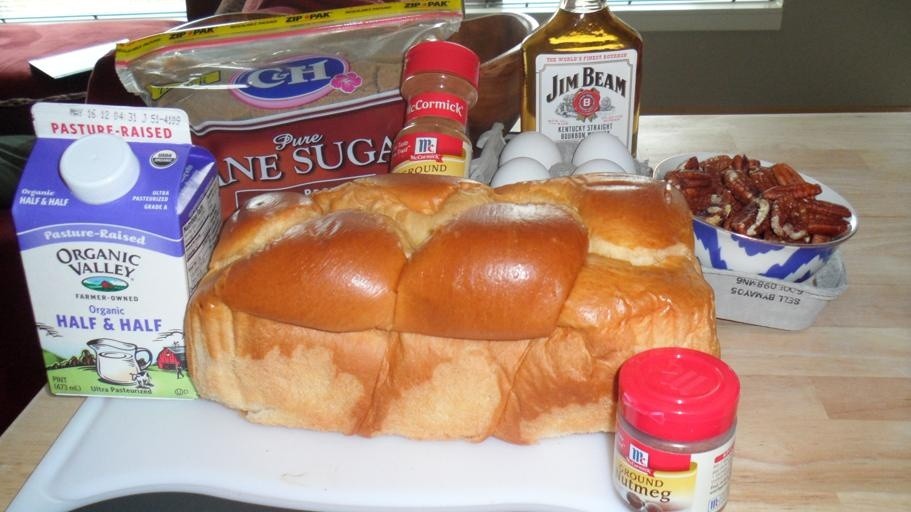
490;131;563;188
572;131;637;176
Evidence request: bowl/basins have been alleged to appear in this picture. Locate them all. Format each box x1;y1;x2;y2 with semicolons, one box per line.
650;151;861;283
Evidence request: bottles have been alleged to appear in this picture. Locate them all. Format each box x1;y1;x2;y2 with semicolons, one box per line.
518;0;647;163
389;39;482;179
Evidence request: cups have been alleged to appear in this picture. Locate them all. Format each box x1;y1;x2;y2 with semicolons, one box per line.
608;346;743;512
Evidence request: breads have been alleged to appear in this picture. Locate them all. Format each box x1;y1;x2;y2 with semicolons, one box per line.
183;172;722;440
